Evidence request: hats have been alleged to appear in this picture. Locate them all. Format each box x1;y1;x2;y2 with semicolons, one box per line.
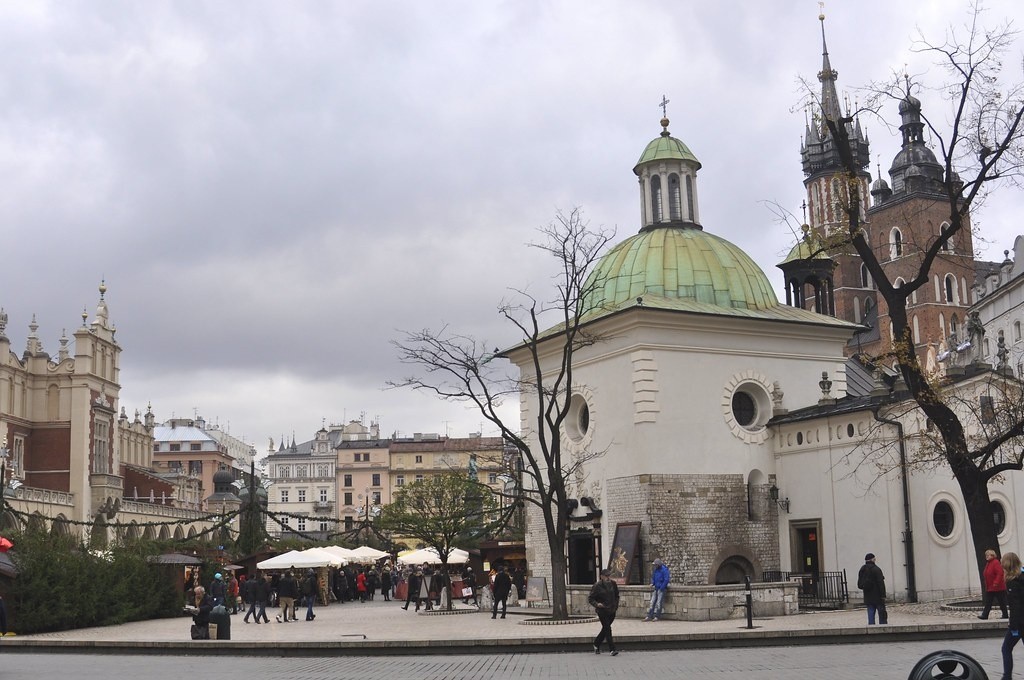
468;567;472;573
652;558;662;566
307;569;314;575
865;553;875;560
340;570;344;574
215;572;222;579
601;569;611;575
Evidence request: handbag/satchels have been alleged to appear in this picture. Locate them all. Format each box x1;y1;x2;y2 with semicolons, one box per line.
191;625;210;640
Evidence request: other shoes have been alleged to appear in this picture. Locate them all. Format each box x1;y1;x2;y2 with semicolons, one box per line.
977;615;988;619
610;650;618;656
401;606;407;610
643;616;652;621
593;644;600;654
472;602;477;604
652;617;658;622
462;600;468;604
306;617;312;621
311;615;316;620
244;617;299;624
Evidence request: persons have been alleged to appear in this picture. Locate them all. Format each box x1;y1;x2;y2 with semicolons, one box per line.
1000;552;1024;680
858;553;888;625
186;568;320;638
643;559;670;621
333;565;526;619
588;569;620;655
978;549;1009;619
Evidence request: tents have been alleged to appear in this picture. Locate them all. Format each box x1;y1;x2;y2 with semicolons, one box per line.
256;544;470;569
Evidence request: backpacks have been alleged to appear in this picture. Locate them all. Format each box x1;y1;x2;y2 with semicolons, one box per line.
302;577;314;596
